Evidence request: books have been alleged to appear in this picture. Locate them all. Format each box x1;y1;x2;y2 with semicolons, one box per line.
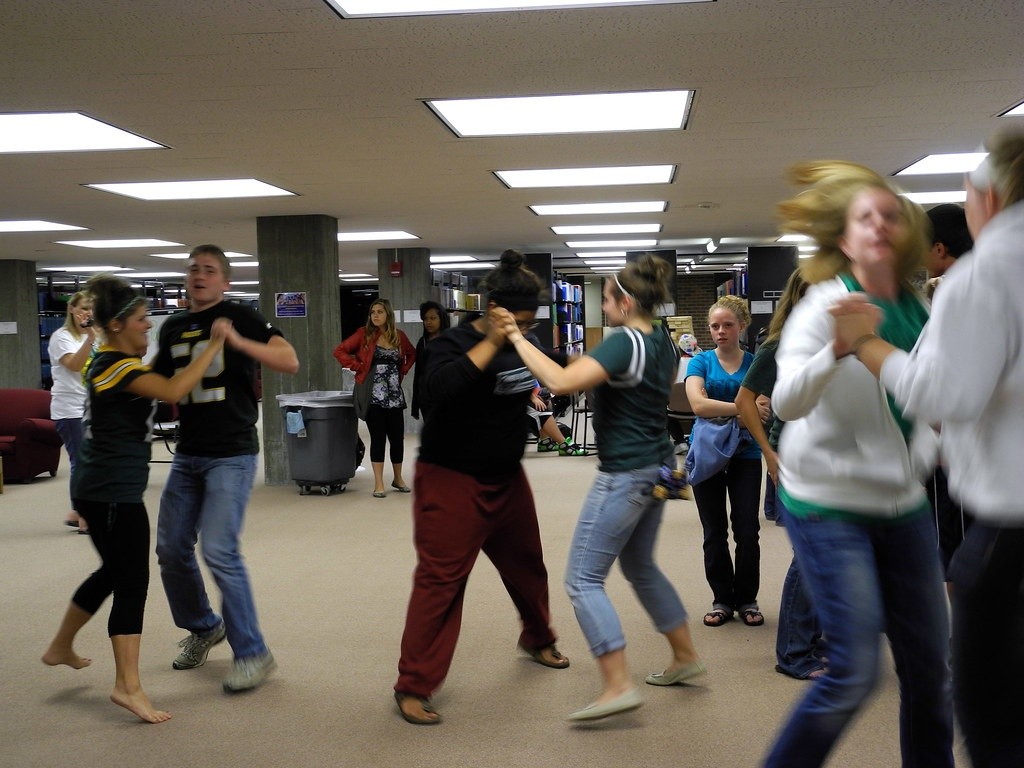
431;268;482;329
717;265;748;300
553;279;583;358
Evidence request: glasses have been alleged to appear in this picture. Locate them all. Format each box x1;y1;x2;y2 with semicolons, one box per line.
517;319;540;330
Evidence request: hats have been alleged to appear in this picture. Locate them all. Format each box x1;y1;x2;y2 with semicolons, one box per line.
679;333;703;357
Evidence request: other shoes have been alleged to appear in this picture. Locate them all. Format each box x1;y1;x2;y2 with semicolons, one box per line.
373;490;386;498
646;661;706;686
570;689;644;721
391;481;411;492
394;691;441;724
520;643;569;668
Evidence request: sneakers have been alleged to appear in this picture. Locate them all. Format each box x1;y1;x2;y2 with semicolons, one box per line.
559;437;588;455
674;443;689;454
172;623;226;670
537;435;571;451
223;653;278;691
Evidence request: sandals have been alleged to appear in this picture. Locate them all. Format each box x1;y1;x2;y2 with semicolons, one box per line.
703;612;733;627
738;610;764;626
774;664;827;680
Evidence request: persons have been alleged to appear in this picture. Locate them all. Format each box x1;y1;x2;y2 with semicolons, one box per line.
411;300;452;424
829;113;1024;768
682;295;773;628
526;392;589;457
277;293;305;304
734;266;829;682
47;291;104;535
333;298;415;497
145;245;301;697
753;159;958;767
919;202;975;305
392;248;585;727
667;332;711;446
494;257;707;722
41;272;232;724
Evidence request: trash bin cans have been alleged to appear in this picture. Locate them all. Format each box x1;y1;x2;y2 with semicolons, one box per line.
276;390;358;495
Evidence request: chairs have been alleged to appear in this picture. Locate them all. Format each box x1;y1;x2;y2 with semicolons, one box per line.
666;382;697;448
0;387;64;483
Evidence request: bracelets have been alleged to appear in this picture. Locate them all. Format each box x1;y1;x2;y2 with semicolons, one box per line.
851;333;879;354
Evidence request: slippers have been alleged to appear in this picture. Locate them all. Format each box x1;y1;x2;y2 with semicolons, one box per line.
67;521;79;527
79;528;90;535
